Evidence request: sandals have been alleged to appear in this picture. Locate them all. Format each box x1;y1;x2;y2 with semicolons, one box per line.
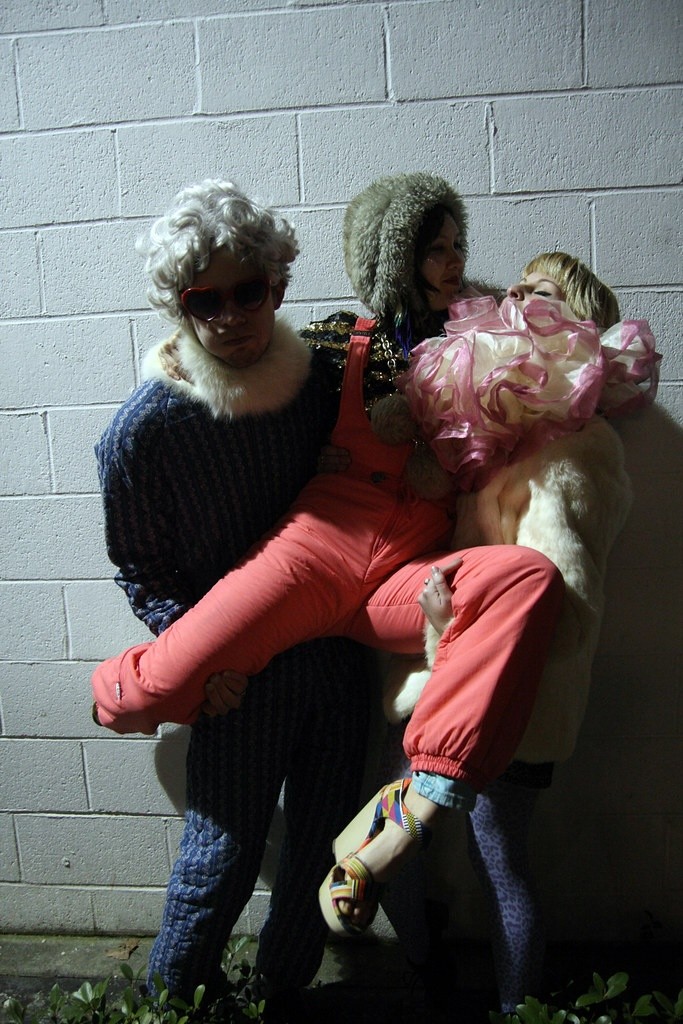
318;776;435;937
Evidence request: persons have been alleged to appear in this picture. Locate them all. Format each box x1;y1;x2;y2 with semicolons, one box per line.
97;178;383;1024
359;254;681;1024
88;171;563;935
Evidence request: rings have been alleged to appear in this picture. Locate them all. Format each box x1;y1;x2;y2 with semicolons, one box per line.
240;689;247;696
424;579;431;585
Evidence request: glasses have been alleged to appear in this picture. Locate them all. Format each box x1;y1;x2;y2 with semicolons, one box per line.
181;275;274;323
